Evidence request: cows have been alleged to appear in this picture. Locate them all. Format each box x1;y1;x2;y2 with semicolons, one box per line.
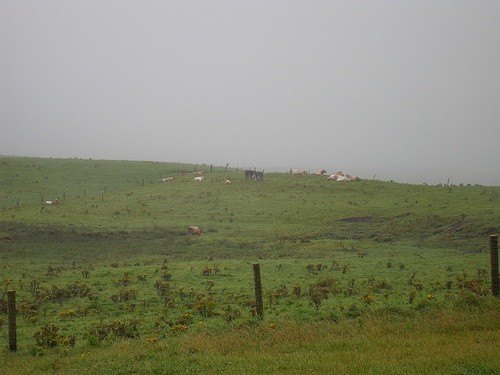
158;176;176;184
193;176;203;181
245;169;265;181
224;179;231;185
327;171;361;182
187;226;201;236
